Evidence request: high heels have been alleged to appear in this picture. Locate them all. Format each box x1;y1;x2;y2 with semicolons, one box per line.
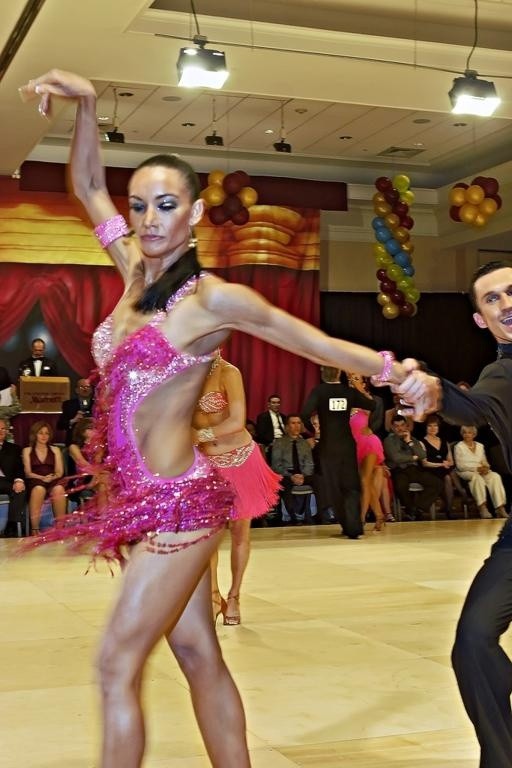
212;591;228;626
373;517;386;531
225;591;241;625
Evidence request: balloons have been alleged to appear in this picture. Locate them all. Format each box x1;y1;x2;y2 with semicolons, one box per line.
370;173;421;319
448;174;502;228
200;169;259;225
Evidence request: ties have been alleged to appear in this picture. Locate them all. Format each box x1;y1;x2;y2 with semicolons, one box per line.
276;415;287;435
399;439;408;468
292;440;301;475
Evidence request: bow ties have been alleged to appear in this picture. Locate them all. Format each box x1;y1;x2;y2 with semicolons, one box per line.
33;357;42;361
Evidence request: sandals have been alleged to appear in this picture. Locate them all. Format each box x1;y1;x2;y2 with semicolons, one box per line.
385;512;395;522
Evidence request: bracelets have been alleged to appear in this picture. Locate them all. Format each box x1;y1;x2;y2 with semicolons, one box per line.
196;426;217;444
371;348;396;386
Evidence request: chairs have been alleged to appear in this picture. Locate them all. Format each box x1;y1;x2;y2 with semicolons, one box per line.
257;441;477;522
0;442;94;537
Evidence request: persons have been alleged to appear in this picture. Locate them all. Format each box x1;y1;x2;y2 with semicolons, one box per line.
383;396;509;520
191;338;283;629
1;338;95;537
16;65;444;768
246;365;395;539
390;259;512;766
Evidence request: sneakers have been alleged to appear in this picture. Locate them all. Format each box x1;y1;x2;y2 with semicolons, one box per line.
495;506;509;518
480;510;492;519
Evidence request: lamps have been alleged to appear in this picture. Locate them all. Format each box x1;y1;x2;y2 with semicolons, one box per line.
105;85;292;155
173;0;232;94
450;0;502;119
